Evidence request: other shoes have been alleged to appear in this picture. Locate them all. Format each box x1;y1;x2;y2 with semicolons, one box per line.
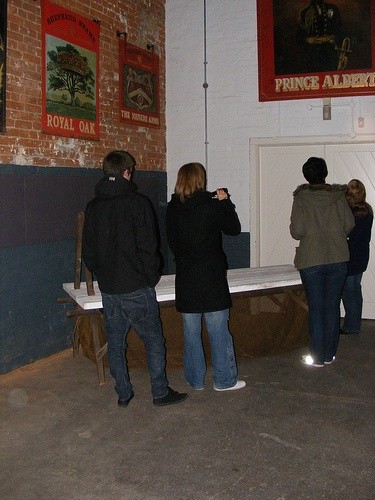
213;379;246;392
153;385;188;406
117;390;135;407
339;329;359;335
300;354;335;368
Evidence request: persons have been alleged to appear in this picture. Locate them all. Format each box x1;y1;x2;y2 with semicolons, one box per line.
339;179;373;335
81;150;189;406
166;162;246;392
290;158;356;368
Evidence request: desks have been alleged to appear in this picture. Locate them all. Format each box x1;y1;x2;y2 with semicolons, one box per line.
58;264;309;385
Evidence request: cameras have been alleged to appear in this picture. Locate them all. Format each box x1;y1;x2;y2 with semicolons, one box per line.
210;188;231;201
